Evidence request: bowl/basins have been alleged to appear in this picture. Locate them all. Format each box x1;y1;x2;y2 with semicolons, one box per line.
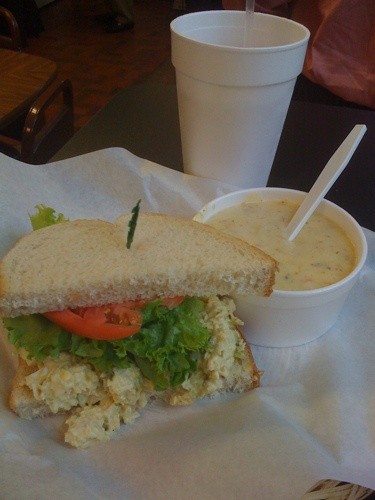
192;187;368;349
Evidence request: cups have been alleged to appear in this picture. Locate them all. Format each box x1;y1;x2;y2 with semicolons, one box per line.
170;10;311;191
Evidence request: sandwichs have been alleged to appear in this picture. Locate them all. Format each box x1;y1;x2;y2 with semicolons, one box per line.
1;197;280;449
